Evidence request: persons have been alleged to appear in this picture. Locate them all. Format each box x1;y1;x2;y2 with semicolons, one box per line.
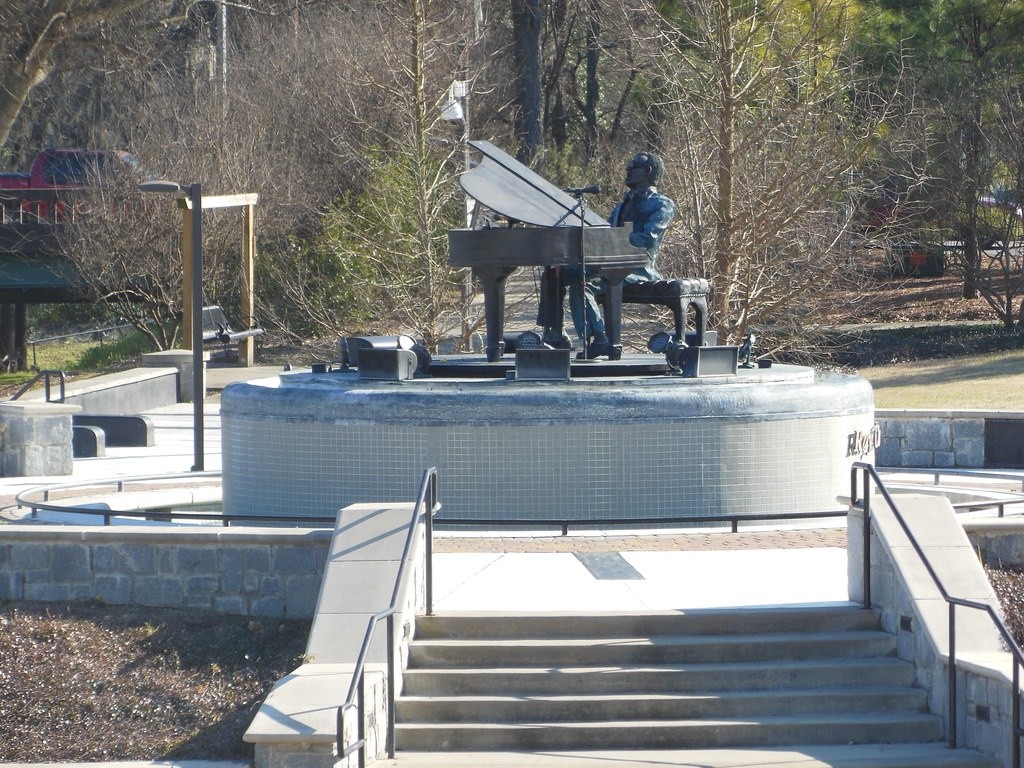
536;150;674;359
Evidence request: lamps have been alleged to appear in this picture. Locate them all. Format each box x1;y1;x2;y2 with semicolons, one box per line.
347;329;741;379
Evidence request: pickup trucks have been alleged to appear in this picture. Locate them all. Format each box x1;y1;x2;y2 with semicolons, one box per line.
0;145;166;225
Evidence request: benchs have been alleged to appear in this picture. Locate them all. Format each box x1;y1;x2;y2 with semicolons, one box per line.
589;277;710;345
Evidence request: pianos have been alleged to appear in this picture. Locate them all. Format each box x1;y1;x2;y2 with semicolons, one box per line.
446;139;649;362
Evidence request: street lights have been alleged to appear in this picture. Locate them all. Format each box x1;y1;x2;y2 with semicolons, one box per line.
137;180;205;472
440;80;475;355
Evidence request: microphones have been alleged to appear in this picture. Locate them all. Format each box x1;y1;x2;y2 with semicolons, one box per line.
567;184;601;194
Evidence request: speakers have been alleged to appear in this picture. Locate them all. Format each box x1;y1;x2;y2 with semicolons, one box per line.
503;332;544;352
666;331;718;347
515;349;571;381
346;335;418;380
680;345;740;378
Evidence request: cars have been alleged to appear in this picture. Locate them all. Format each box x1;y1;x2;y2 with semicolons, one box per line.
852;191;1024;250
